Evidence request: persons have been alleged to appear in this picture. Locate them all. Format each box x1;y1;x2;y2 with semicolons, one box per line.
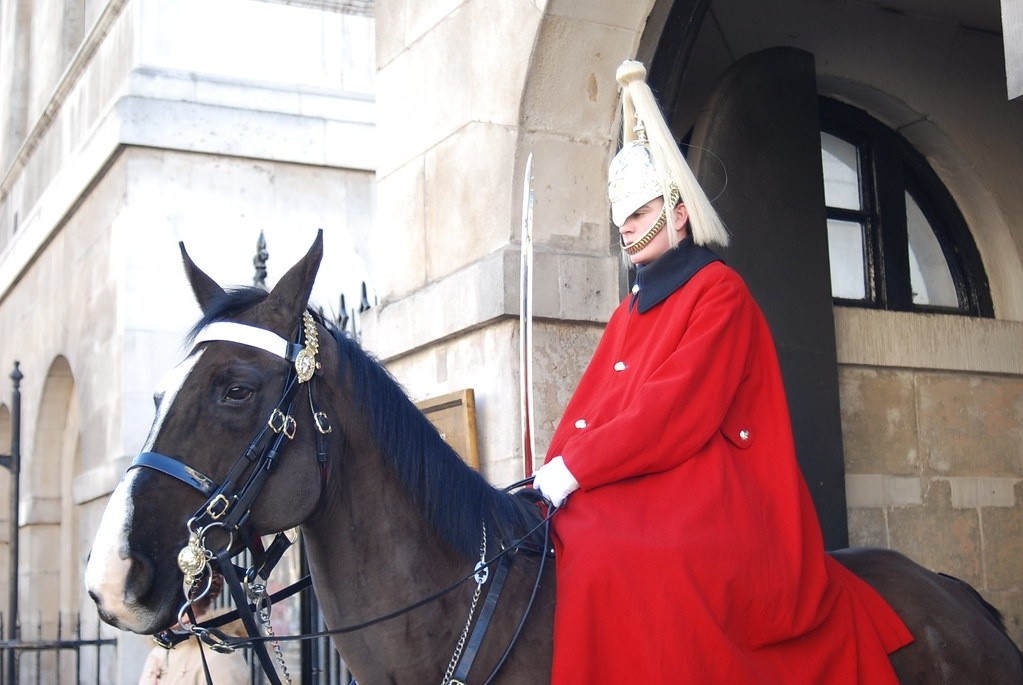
530;139;801;685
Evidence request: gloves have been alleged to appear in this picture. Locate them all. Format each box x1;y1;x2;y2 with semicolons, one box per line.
531;455;581;509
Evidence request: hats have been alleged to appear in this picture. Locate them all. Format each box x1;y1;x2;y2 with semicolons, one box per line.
608;62;732;255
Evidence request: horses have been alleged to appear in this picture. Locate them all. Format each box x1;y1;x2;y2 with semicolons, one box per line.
84;227;1023;685
